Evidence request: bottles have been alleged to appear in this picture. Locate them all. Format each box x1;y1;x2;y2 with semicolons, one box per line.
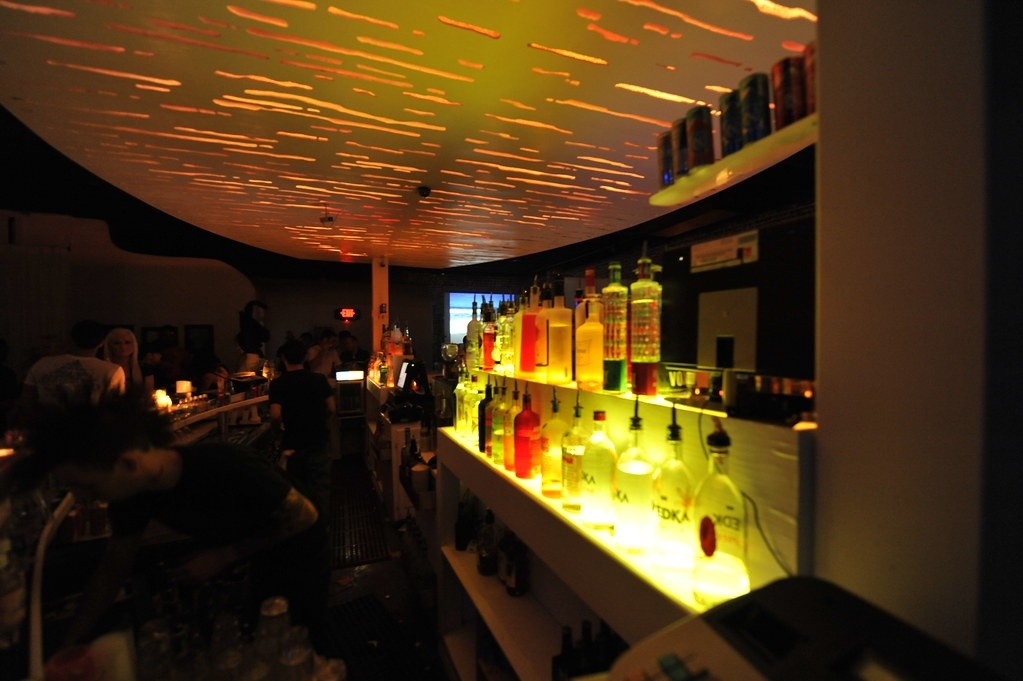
368;323;415;385
455;363;751;600
401;426;437;517
551;617;627;681
0;537;25;651
464;254;661;400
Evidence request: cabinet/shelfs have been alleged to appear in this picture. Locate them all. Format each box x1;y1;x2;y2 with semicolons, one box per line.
375;105;819;681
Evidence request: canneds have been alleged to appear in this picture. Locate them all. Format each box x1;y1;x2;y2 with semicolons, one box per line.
656;40;816;190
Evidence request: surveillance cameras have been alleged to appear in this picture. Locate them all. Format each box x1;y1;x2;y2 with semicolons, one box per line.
416;185;434;198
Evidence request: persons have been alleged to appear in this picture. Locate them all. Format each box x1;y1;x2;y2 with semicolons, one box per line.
0;327;371;656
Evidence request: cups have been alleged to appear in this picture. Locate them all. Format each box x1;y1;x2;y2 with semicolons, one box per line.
4;428;27;449
441;342;458;363
722;369;755;410
151;389;168;411
175;380;192;404
47;594;312;681
